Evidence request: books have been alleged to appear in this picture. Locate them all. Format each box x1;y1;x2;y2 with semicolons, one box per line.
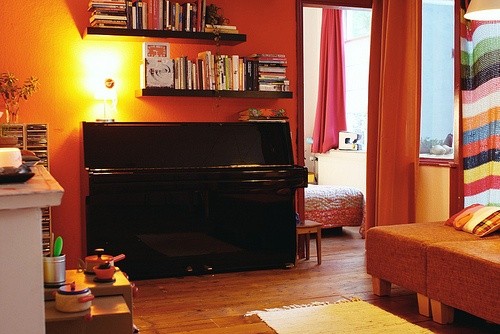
174;50;290;91
88;0;239;35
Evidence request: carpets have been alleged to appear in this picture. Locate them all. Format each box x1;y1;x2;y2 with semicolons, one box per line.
244;298;434;334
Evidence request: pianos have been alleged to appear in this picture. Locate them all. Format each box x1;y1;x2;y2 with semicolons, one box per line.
83;120;309;283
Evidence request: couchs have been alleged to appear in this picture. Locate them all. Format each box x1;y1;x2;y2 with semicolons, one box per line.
365;221;500;325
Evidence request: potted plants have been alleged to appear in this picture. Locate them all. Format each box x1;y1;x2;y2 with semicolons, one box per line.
0;72;39;124
43;233;66;284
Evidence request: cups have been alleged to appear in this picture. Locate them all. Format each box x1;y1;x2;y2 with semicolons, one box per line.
0;147;22;168
93;266;116;279
43;253;65;283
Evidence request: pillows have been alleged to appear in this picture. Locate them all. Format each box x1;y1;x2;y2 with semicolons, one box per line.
308;172;315;184
445;203;500;238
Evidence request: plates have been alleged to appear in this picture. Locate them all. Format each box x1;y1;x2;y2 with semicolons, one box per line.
93;277;114;282
84;271;96;275
0;167;35;183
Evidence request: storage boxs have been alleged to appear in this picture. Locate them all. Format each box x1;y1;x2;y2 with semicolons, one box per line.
46;297;131;334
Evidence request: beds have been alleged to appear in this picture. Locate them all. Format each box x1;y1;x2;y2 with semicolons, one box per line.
304;184;364;233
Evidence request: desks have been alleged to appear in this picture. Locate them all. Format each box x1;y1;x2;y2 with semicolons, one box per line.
44;267;132;334
0;160;64;334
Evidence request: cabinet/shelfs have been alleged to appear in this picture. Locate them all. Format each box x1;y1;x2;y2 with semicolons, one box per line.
81;0;294;99
0;124;54;258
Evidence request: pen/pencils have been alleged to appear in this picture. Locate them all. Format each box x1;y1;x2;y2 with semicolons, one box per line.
48;232;64;256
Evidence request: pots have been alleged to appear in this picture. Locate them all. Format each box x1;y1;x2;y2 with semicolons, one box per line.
84;249;125;275
53;281;94;312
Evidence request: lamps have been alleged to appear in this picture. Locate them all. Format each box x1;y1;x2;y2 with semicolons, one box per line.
84;50;122;121
79;48;82;49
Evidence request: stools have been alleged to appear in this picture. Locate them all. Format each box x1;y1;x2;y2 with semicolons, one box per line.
297;220;323;266
